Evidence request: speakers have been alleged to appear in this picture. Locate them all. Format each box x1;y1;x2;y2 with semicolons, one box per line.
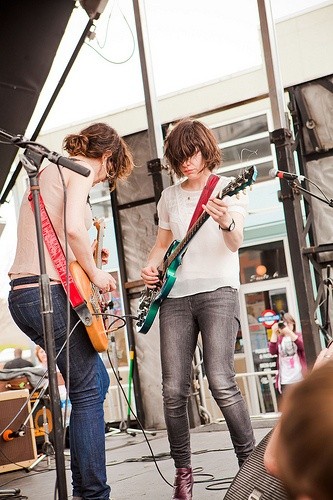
223;427;288;500
0;388;38;474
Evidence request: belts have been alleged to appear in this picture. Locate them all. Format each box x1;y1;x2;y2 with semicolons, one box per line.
12;281;62;290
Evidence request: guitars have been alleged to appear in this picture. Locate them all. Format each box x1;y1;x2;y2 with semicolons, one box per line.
135;164;257;334
71;215;108;352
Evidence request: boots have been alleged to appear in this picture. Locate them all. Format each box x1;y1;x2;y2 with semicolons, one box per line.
173;468;193;500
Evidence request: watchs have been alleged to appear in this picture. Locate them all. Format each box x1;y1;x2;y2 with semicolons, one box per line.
219;219;235;232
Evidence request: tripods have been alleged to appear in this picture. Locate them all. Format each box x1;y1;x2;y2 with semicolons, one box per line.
15;379;56;473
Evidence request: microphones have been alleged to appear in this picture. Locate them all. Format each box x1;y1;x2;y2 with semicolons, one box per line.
3;429;26;441
269;168;307;180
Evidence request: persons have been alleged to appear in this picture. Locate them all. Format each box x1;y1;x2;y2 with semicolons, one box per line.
6;122;135;500
264;359;333;500
3;348;35;370
33;346;61;373
267;311;309;407
142;120;255;500
